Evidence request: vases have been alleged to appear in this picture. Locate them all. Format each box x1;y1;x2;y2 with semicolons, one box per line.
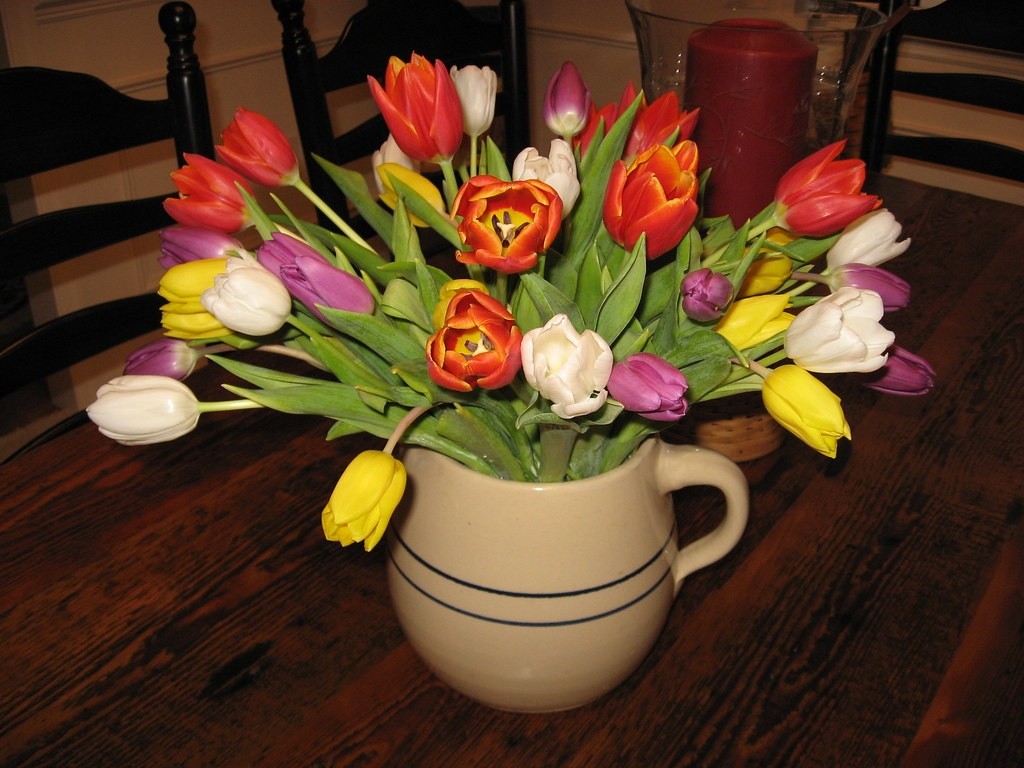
380;429;752;712
625;0;888;467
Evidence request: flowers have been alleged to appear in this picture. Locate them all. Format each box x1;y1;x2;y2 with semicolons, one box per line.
84;50;938;553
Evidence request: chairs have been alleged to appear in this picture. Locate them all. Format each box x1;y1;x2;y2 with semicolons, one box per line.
0;0;245;438
272;0;532;278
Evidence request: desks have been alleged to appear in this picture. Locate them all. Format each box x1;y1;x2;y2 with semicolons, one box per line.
0;159;1024;768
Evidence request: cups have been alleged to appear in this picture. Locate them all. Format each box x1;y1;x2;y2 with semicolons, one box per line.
625;0;888;232
